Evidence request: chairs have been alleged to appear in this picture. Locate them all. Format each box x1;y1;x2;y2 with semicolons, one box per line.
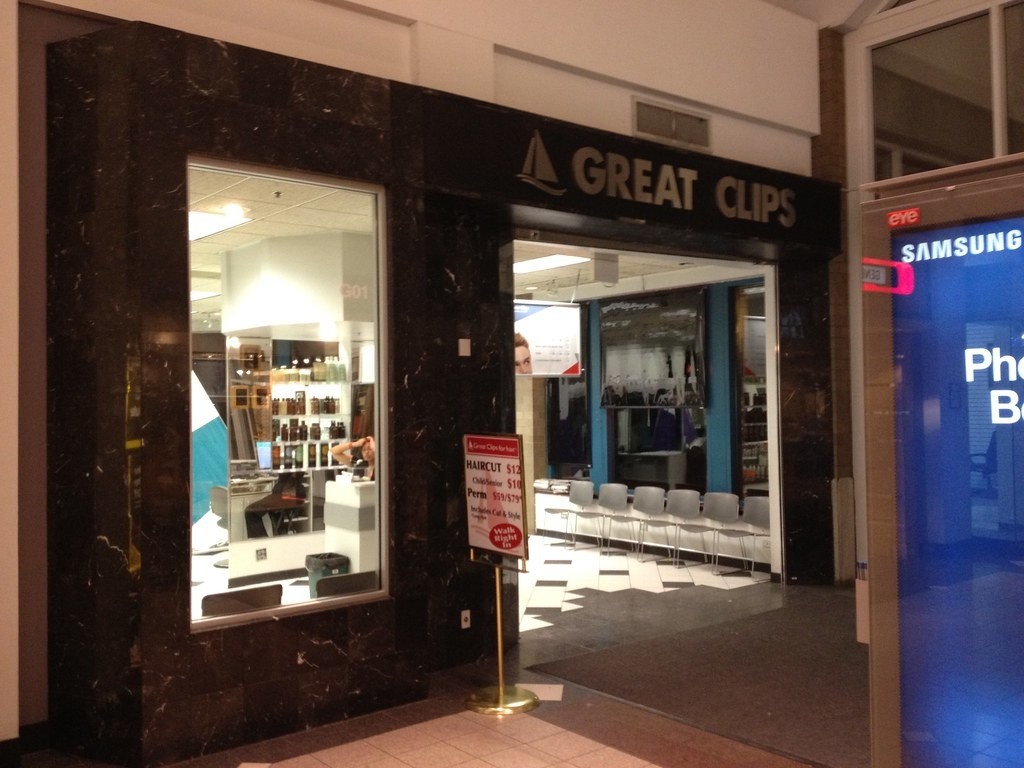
201;583;283;616
210;485;228;568
314;570;376;598
548;476;772;580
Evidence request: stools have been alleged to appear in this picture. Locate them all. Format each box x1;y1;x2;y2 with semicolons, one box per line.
271;505;301;534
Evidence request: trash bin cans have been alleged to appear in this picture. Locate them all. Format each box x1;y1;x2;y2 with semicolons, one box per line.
305;552;350;599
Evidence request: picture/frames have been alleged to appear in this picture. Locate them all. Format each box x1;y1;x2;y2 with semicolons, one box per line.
240;344;263;373
231;386;249;410
251;385;268;409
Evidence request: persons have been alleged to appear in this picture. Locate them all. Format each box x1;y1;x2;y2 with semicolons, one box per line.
243;461;307;539
329;436;375;482
514;331;533;374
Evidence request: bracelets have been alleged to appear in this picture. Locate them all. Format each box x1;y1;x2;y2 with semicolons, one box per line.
348;442;352;449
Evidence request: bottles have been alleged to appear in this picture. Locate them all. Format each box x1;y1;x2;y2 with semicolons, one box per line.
272;396;346;470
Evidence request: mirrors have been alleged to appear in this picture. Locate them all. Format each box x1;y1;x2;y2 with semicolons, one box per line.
228;322;353;588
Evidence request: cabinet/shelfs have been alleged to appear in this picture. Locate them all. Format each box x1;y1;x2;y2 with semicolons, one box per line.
268;374;349;475
229;491;274;542
